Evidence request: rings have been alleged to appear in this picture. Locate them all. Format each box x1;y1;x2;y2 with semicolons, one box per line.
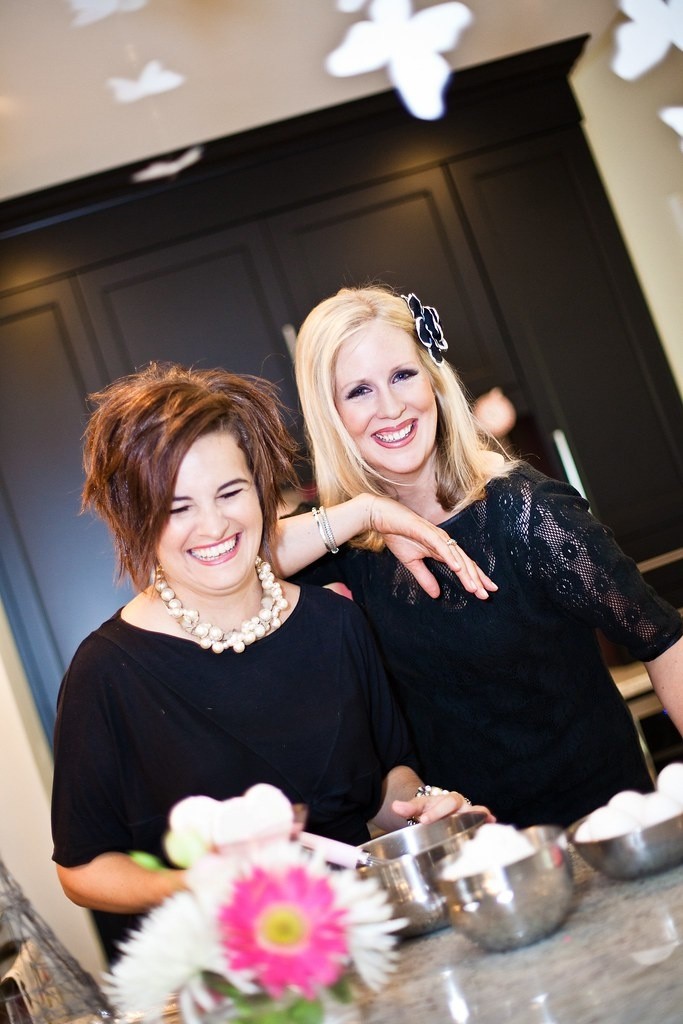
446;539;456;545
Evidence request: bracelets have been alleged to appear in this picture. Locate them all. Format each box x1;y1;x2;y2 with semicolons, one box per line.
312;506;338;553
408;786;472;826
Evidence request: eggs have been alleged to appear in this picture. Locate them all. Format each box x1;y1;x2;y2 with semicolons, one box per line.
575;762;683;844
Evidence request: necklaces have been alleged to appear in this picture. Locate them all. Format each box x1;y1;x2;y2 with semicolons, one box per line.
155;556;288;654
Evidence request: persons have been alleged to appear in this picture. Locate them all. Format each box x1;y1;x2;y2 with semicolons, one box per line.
53;365;495;987
263;289;683;830
449;357;515;448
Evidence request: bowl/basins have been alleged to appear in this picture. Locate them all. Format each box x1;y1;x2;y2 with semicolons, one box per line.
342;811;488;935
567;792;681;880
431;826;573;952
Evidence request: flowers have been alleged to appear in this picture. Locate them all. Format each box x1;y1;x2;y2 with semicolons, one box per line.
96;848;407;1024
401;293;448;366
